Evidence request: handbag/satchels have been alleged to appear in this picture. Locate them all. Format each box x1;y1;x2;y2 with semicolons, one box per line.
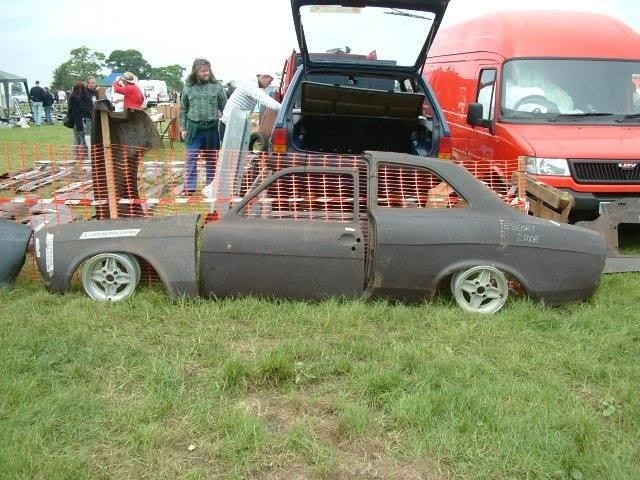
63;112;73;128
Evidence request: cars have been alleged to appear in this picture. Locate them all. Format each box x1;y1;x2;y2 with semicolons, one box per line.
11;91;30;105
264;0;455;211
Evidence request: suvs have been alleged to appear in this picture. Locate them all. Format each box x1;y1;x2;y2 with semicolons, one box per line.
267;46;398;117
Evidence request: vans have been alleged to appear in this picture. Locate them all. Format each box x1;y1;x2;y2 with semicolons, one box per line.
415;6;640;219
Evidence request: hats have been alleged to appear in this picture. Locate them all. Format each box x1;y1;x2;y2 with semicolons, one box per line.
259;69;274;78
119;72;138;83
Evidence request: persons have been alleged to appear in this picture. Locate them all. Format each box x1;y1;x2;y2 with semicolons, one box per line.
29;59;281;194
